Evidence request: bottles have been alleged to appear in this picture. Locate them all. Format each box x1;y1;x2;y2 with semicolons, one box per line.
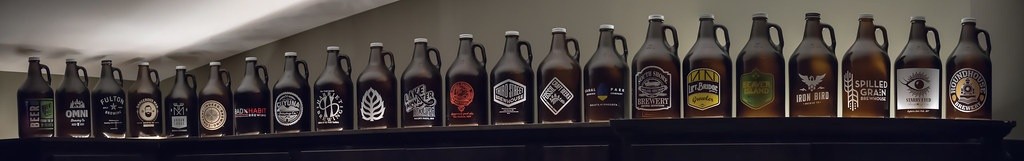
314;46;354;131
583;24;630;123
537;28;582;123
683;13;732;118
736;12;786;117
894;16;943;119
841;14;891;118
631;15;681;119
788;14;838;117
17;56;234;138
445;33;488;127
234;57;271;136
400;37;443;128
946;17;992;120
490;31;535;125
356;43;398;129
273;52;311;134
